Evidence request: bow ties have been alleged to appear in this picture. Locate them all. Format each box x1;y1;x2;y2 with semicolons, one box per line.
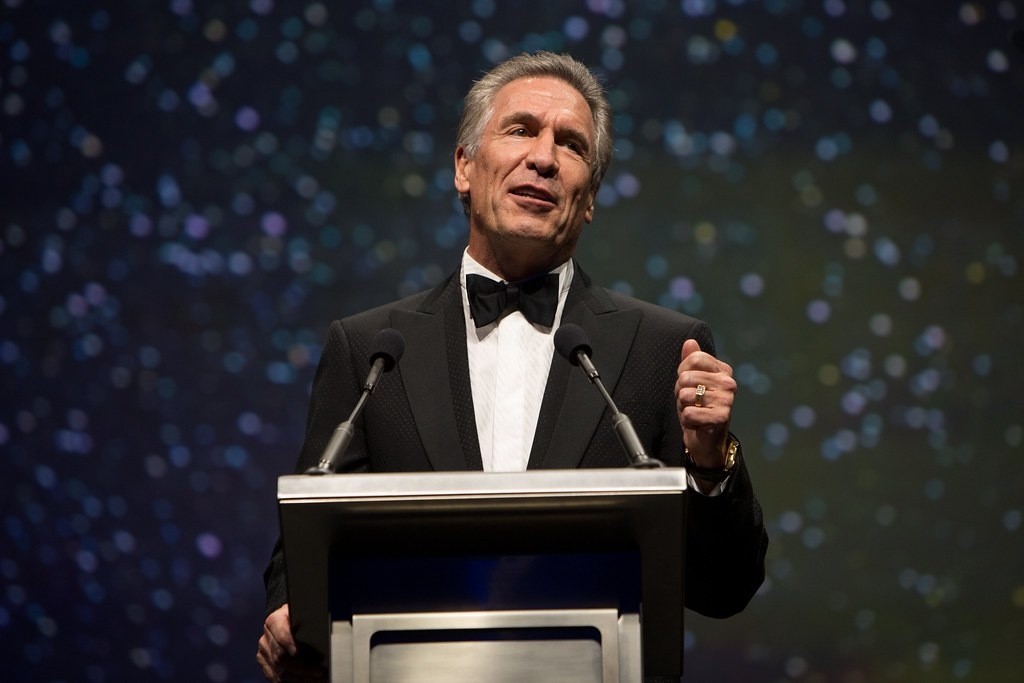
466;273;559;328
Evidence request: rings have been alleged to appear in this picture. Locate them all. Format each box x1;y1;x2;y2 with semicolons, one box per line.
695;385;706;407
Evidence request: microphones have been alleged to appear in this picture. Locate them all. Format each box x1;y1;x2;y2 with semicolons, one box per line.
553;323;664;468
304;329;405;476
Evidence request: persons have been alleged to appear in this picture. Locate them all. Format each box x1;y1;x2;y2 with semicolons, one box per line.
254;47;771;683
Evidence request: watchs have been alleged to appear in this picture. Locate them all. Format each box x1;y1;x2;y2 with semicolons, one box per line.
683;432;740;483
